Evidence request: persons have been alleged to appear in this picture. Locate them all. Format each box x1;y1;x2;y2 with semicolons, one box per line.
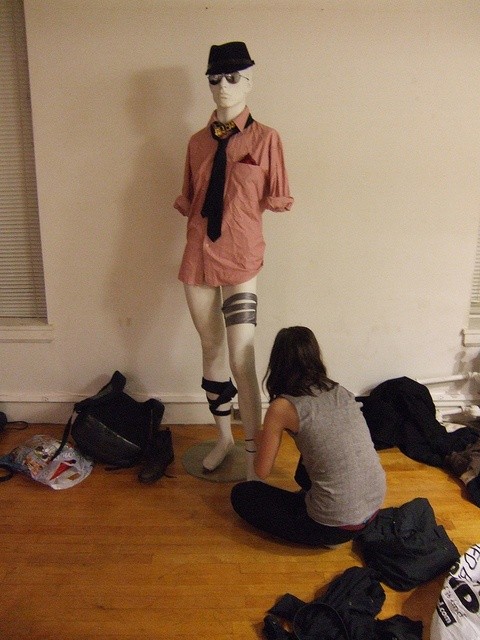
173;42;294;482
230;326;388;547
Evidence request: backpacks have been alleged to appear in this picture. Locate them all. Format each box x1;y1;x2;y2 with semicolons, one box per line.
48;371;175;484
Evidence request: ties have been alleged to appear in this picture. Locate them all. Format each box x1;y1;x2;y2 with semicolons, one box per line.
200;113;255;242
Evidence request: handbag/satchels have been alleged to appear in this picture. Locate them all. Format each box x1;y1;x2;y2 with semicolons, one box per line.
0;433;93;489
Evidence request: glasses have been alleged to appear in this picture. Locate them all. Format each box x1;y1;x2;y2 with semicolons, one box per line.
207;71;249;85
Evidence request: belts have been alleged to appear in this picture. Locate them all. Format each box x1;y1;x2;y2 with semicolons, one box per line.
341;512;378;530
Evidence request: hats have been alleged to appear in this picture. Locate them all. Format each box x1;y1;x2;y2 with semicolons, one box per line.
205;42;255;75
